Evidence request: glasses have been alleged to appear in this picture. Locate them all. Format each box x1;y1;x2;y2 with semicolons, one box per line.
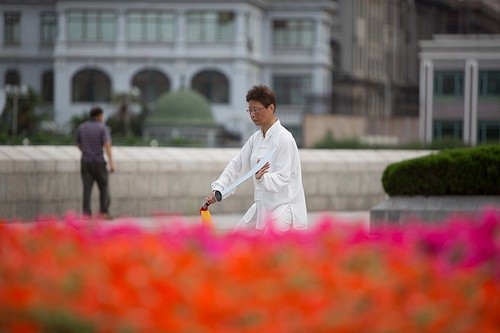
247;105;268;114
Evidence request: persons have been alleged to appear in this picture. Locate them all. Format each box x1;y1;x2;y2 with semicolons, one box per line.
206;84;307;235
75;106;115;220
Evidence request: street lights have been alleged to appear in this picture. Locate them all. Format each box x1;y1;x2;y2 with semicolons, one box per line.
5;84;27;145
111;87;142;139
